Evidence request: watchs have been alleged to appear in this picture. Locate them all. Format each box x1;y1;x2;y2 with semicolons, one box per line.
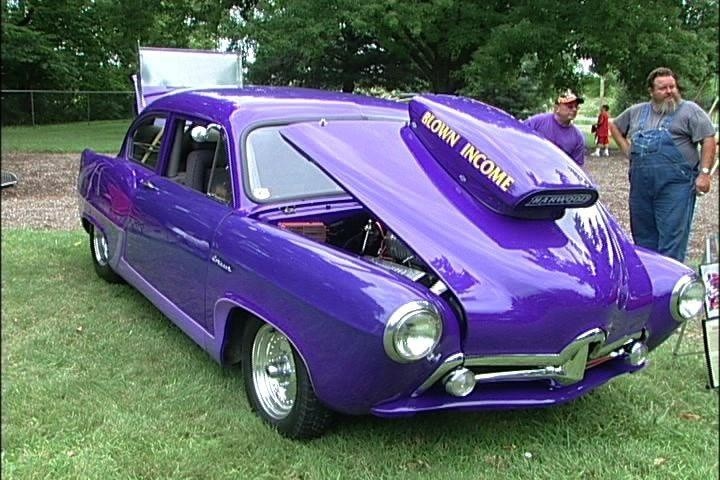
698;165;712;175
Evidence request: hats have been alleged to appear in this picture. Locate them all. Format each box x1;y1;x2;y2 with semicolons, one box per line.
555;94;584;104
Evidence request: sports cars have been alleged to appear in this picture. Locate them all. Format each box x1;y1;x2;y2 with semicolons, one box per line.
70;81;709;446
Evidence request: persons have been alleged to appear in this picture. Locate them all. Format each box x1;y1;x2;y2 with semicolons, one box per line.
589;105;611;160
609;68;717;262
521;93;584;166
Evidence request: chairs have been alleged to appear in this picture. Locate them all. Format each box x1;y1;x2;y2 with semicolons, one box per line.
132;125;228;200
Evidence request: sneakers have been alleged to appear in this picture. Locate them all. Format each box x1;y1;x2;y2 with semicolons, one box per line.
590;152;600;157
602;152;609;157
